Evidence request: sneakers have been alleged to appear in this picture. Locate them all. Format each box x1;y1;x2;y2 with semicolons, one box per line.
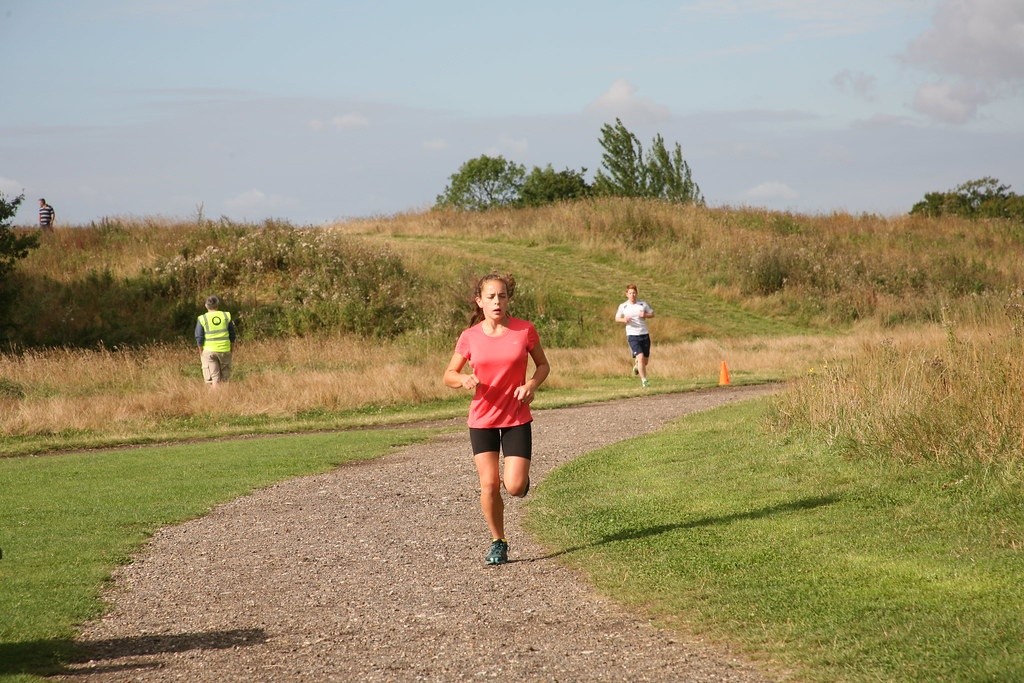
485;539;511;565
518;475;531;498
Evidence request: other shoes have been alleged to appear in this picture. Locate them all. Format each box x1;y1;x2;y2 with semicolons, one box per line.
632;362;639;376
642;380;651;388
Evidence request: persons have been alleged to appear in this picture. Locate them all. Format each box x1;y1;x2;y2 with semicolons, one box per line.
443;273;550;564
194;295;236;385
615;284;654;387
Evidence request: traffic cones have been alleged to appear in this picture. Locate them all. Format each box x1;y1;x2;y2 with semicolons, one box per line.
717;361;731;385
